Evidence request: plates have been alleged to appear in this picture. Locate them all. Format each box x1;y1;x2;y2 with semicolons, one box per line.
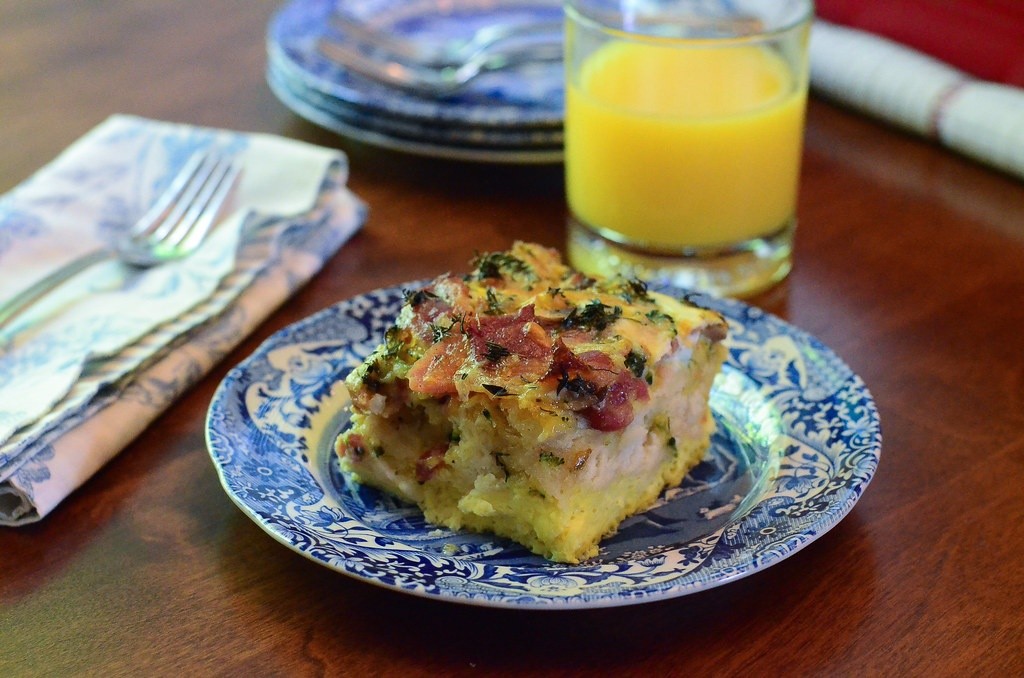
205;267;881;609
261;1;567;168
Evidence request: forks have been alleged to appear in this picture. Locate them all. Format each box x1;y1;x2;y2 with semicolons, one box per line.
317;33;564;101
321;13;562;67
0;149;242;336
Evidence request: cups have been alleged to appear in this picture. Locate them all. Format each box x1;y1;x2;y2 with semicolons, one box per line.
563;0;818;300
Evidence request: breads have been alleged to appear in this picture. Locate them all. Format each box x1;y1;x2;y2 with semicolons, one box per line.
333;239;730;564
